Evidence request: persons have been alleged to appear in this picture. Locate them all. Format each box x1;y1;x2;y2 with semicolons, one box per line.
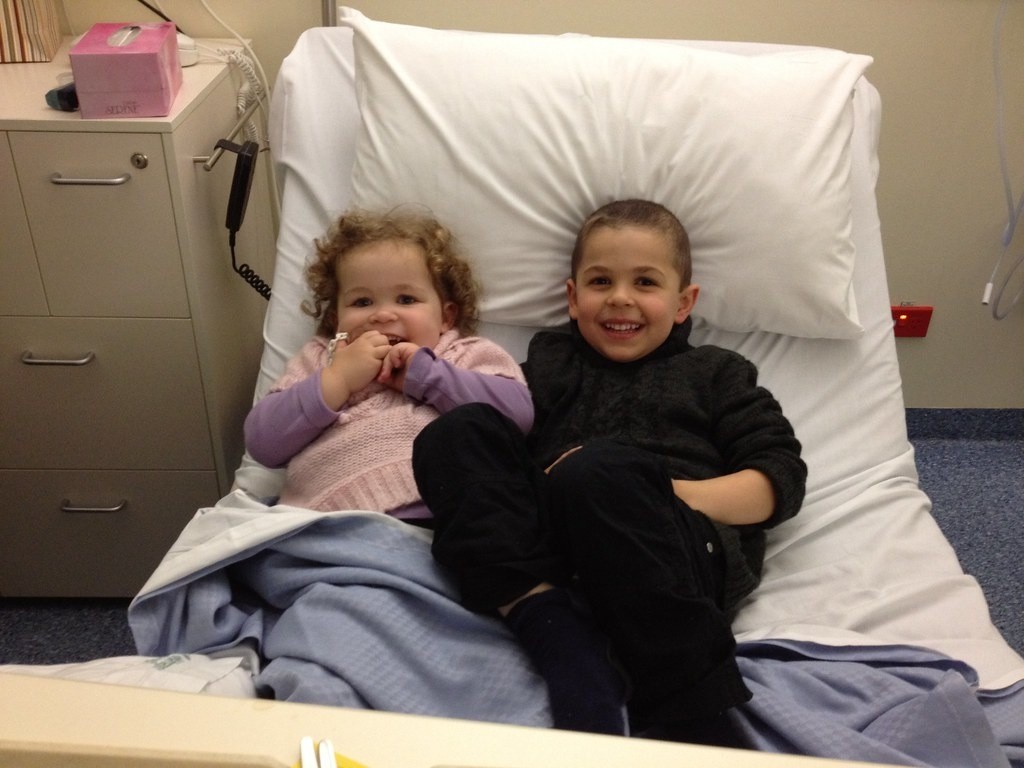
243;205;534;529
412;198;809;742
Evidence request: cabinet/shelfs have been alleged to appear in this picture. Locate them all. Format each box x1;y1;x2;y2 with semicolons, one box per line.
0;34;277;603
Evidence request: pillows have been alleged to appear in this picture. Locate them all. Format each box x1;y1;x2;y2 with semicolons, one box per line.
340;5;876;343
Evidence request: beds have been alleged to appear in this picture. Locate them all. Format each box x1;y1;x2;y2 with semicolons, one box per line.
3;26;1024;765
